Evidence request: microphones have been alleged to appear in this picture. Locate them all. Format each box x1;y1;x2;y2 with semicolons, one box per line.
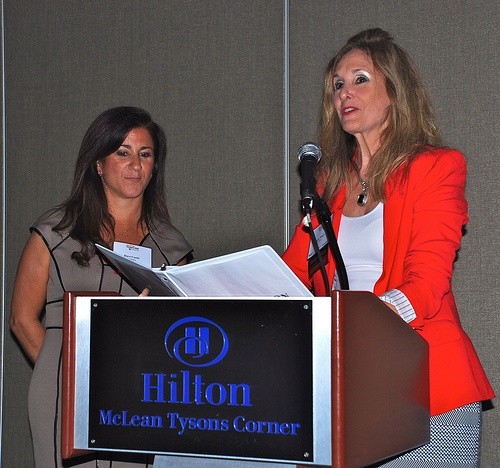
297;142;322;213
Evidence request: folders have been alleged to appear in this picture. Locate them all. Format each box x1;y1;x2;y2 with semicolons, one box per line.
95;243;313;297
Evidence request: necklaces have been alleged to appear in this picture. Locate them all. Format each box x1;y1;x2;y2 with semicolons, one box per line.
352;157;372;207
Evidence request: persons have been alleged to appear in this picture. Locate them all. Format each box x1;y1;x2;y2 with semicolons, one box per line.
278;29;495;468
8;107;195;468
